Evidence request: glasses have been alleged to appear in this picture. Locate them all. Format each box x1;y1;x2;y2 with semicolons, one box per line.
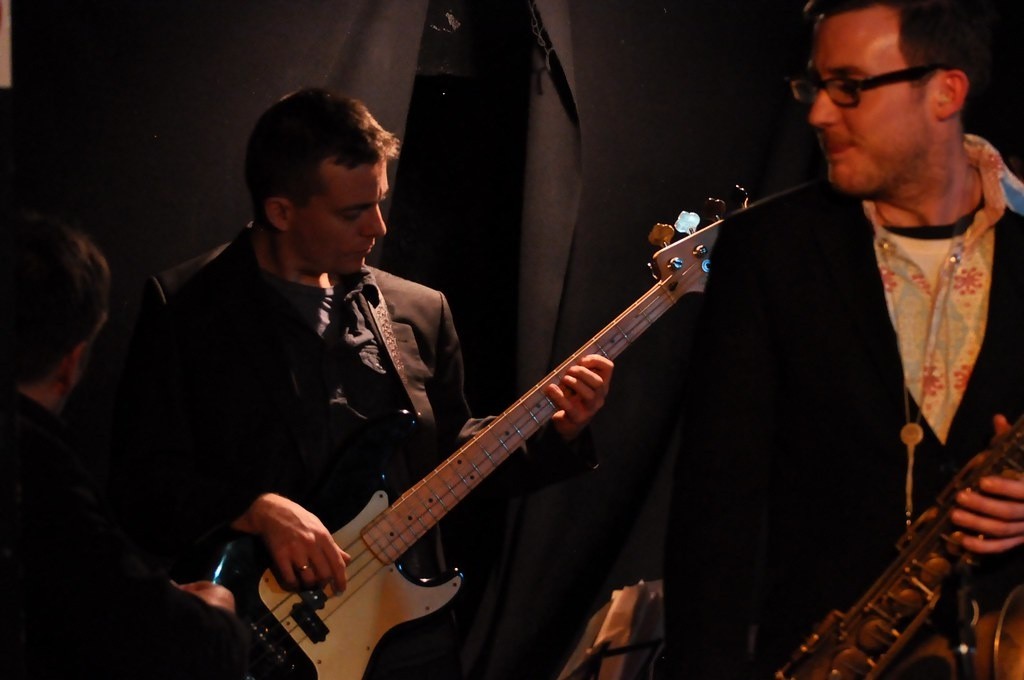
790;61;962;109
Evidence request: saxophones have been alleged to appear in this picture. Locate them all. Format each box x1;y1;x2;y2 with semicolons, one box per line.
772;414;1024;680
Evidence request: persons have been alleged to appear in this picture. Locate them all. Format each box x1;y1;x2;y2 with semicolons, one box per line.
657;0;1024;680
101;86;618;680
0;199;253;680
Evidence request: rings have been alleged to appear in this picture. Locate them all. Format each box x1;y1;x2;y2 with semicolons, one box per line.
298;566;310;571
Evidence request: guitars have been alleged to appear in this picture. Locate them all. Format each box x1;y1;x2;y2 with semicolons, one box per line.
235;183;751;680
